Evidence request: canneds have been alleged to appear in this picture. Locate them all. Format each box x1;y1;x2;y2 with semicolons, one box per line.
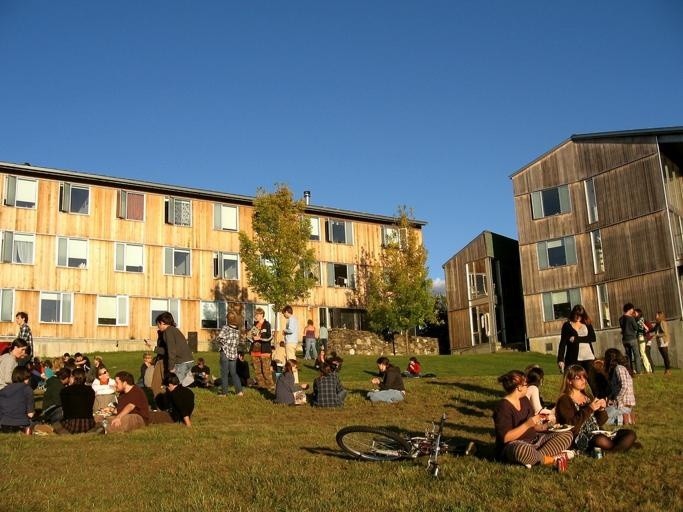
595;447;601;459
557;456;565;474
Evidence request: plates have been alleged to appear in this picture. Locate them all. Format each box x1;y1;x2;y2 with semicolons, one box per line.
549;424;574;433
592;430;615;437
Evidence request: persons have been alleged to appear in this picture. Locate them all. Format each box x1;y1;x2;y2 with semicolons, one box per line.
493;303;671;473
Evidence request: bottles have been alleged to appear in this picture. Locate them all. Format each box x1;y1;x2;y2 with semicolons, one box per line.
464;441;476;457
101;415;108;436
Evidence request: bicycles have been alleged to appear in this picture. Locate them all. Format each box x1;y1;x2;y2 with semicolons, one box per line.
335;412;478;480
